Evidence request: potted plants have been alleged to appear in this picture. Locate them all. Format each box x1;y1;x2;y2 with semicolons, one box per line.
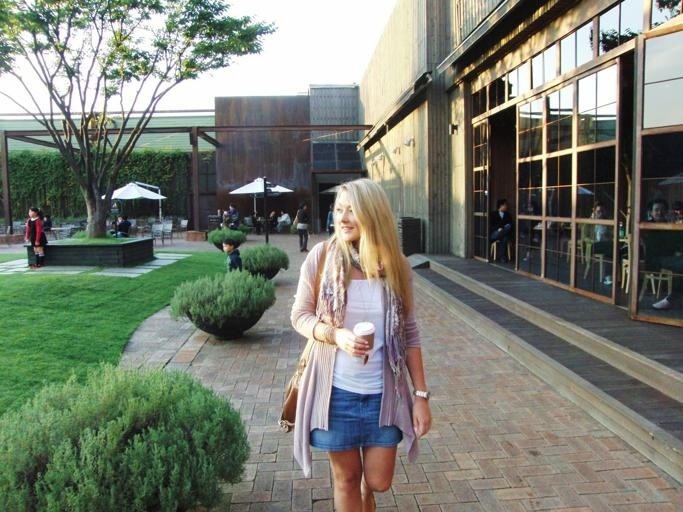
237;244;291;281
167;268;277;340
208;227;248;252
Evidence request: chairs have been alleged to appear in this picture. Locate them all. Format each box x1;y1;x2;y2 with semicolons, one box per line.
0;215;189;248
565;216;683;302
208;214;293;236
490;239;511;262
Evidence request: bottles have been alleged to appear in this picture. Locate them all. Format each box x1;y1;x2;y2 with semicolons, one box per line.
618;221;624;238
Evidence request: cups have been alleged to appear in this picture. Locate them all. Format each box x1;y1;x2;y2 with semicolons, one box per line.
354;320;377;359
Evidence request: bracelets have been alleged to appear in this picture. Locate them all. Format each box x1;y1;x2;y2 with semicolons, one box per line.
411;388;429;400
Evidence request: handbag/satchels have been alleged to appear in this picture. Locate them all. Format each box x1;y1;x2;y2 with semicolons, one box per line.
277;368;302;433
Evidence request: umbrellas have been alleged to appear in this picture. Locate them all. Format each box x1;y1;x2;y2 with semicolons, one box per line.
228;176;293;221
102;182;165;226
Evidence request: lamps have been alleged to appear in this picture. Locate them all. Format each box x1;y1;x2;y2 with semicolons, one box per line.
370;138;412;167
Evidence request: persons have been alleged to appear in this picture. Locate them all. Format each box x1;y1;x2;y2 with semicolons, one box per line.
293;203;311;251
488;197;515;265
215;201;292;271
43;215;52;229
640;198;683;273
580;202;613;287
651;281;681;310
290;177;429;511
114;215;132;238
325;203;338;236
22;205;45;268
